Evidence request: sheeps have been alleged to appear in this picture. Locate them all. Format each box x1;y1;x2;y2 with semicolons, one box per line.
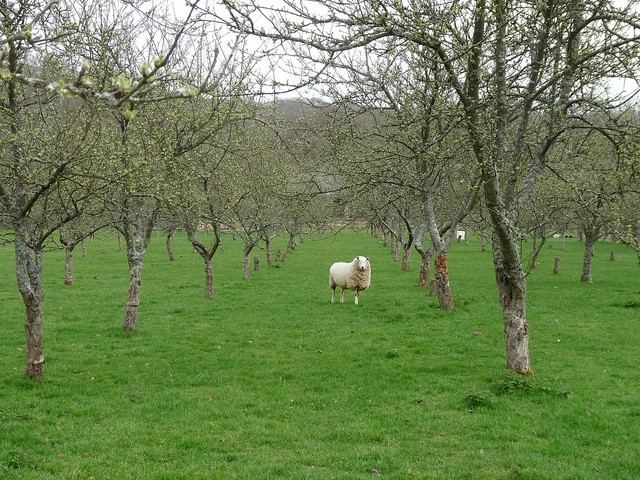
328;256;372;305
